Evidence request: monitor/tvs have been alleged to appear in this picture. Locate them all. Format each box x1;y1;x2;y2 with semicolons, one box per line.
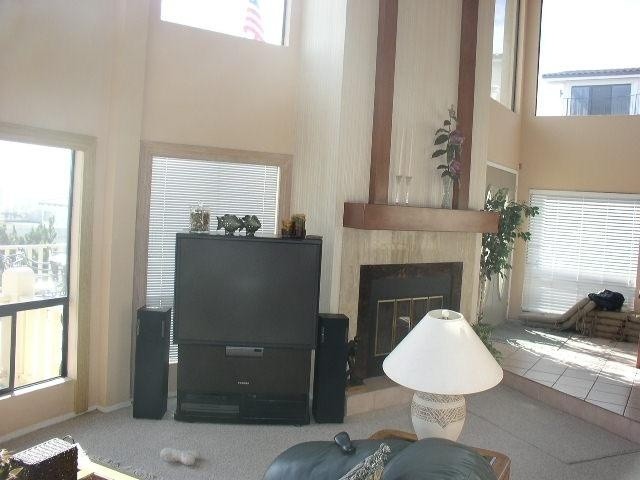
173;233;321;349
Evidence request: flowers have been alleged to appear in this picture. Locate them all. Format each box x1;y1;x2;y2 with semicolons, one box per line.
431;105;466;180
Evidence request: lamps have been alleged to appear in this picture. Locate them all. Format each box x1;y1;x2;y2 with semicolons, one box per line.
382;309;504;443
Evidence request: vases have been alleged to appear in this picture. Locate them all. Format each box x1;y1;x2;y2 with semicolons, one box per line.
440;175;453;209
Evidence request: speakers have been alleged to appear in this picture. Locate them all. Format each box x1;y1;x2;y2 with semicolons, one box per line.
313;313;350;425
133;305;170;418
